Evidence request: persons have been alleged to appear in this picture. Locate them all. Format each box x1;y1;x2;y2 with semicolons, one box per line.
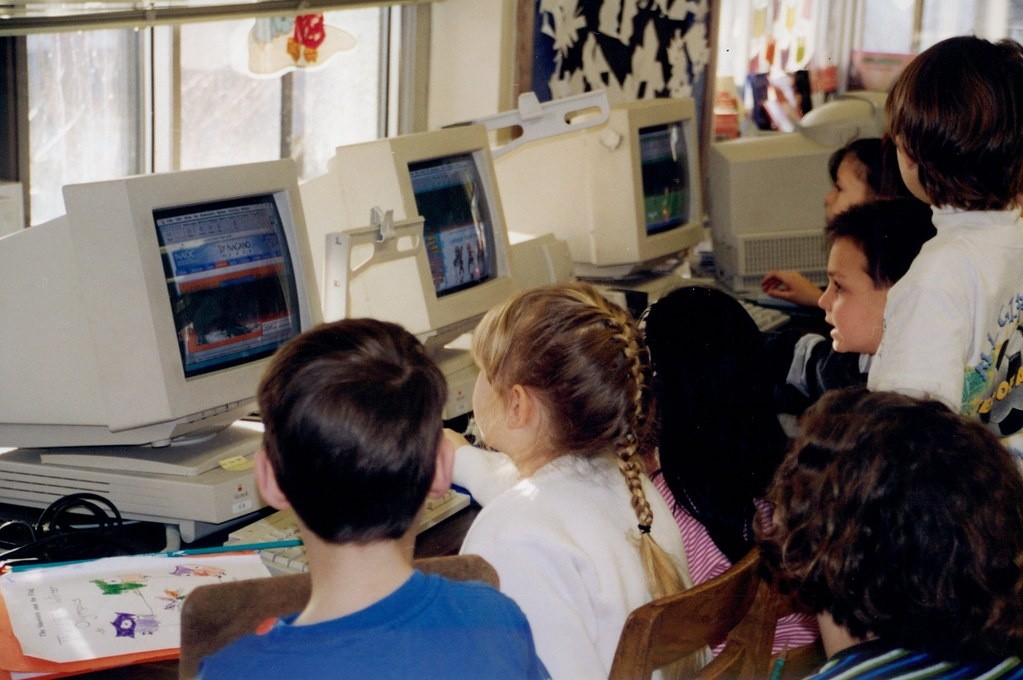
639;285;821;680
765;385;1023;680
761;138;937;442
442;283;714;680
867;35;1023;478
194;317;554;680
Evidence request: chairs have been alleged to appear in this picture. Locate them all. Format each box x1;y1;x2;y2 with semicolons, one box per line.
608;551;781;680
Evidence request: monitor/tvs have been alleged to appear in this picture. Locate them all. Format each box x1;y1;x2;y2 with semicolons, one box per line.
709;90;893;292
0;159;323;450
297;124;522;378
492;96;715;320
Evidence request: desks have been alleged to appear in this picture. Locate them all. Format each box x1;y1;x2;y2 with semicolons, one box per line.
0;285;859;680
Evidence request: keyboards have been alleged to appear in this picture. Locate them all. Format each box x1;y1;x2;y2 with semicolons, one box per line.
224;486;470;578
737;299;780;329
